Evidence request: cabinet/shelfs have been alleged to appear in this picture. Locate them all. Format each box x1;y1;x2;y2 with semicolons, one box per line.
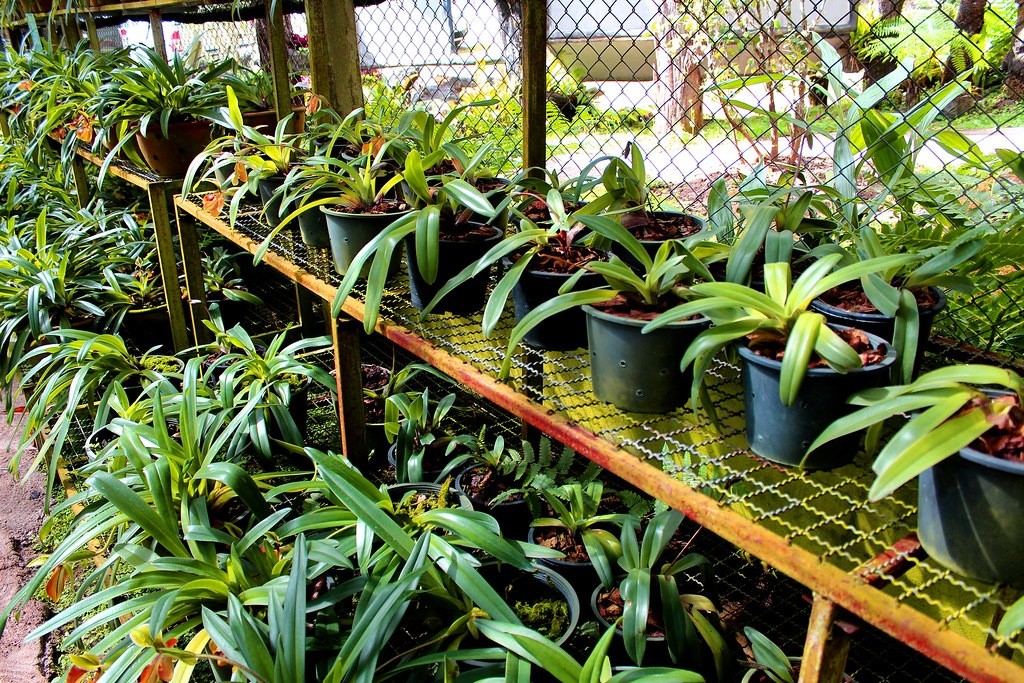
173;3;1024;683
0;0;297;364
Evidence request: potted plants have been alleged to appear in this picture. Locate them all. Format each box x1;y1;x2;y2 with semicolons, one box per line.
0;0;1024;683
